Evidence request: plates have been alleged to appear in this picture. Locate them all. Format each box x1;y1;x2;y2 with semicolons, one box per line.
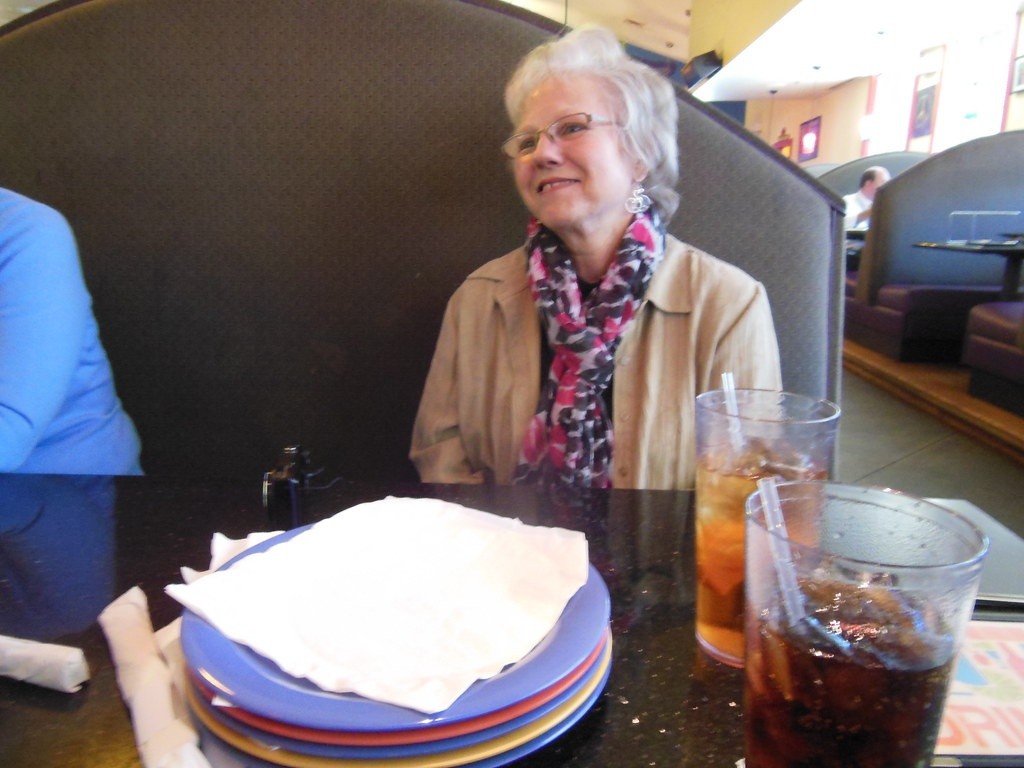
182;522;616;768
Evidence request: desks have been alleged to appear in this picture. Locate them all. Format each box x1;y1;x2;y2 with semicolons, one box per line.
911;238;1023;299
0;473;1024;768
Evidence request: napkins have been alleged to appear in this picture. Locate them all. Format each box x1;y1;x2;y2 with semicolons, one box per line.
1;495;591;768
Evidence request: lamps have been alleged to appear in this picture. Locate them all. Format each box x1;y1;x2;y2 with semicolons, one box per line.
679;49;722;90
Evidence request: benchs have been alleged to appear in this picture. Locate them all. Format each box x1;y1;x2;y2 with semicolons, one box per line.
874;283;1003;369
962;303;1024;407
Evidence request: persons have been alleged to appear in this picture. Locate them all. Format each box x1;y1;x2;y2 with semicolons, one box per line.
842;166;891;230
0;473;117;642
410;25;782;491
0;186;146;476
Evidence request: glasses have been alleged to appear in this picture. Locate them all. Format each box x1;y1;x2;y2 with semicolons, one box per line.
501;113;625;159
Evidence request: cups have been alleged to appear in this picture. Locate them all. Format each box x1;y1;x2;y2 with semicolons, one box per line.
694;386;843;668
744;480;989;767
